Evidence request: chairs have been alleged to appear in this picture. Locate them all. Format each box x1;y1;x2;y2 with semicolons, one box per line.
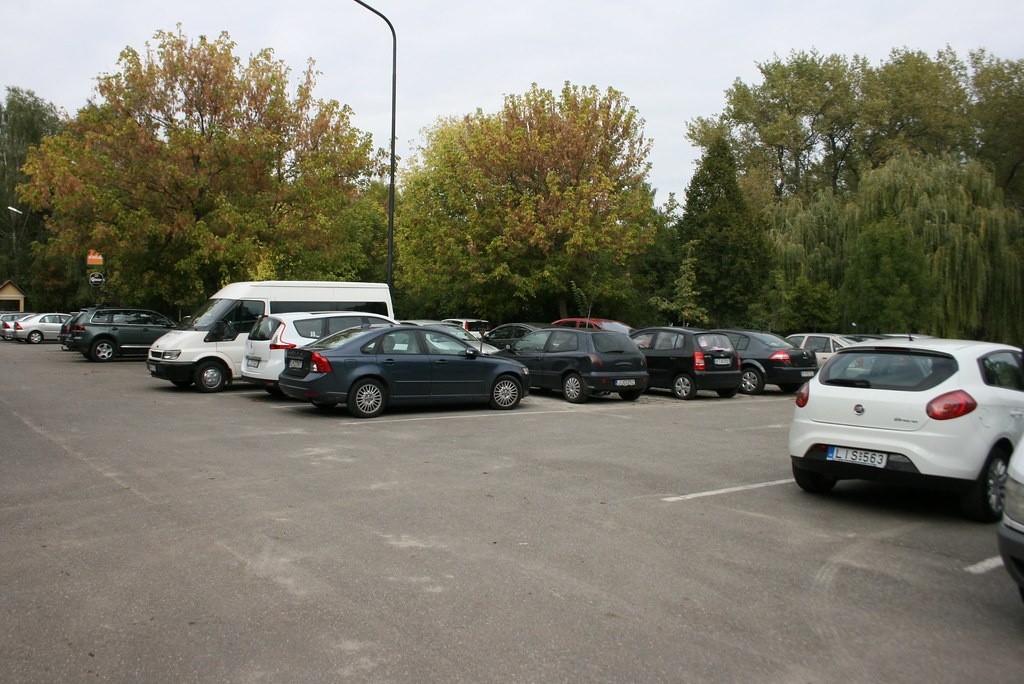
381;336;396;354
657;337;673;350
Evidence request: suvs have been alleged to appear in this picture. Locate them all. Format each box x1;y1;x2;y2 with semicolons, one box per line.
241;310;452;400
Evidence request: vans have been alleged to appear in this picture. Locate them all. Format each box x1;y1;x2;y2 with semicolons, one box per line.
147;280;395;394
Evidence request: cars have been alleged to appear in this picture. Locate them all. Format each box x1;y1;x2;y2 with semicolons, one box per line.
0;307;185;364
490;326;650;403
627;325;743;400
711;328;821;396
278;321;531;419
789;338;1024;524
785;333;873;380
405;317;652;359
